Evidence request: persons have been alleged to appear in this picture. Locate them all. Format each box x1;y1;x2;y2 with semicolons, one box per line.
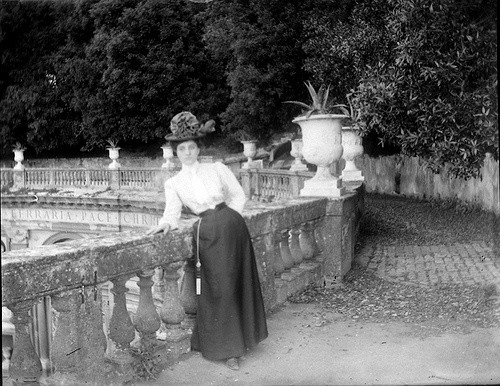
146;111;269;367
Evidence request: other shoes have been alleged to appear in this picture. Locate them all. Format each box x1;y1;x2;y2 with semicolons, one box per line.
226;356;240;370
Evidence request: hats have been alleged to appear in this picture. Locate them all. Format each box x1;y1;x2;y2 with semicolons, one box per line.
164;111;216;141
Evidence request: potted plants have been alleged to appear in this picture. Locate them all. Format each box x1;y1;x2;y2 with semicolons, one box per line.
241;135;263;169
282;80;349;198
12;142;27;168
161;143;176;168
106;138;122;168
336;94;364;181
290;130;309;171
